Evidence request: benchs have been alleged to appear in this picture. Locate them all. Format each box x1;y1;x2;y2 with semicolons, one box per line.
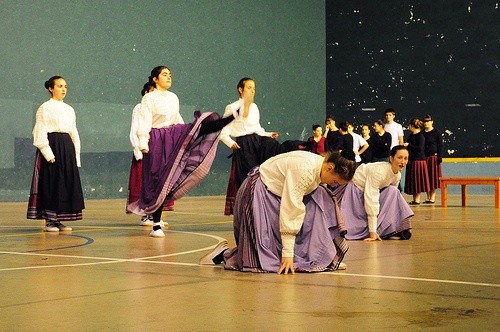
438;176;500;210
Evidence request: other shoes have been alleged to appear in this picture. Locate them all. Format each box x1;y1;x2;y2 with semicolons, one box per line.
409;201;420;205
54;221;72;230
390;237;400;239
149;217;168;225
45;222;59;232
423;201;434;204
150;222;165;237
232;92;250;119
338;262;346;270
199;241;229;265
140;217;153;226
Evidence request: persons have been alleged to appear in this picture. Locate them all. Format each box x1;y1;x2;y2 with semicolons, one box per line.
27;76;85;231
199;144;357;275
127;66;253;238
282;108;443;205
333;145;415;242
219;77;280;215
